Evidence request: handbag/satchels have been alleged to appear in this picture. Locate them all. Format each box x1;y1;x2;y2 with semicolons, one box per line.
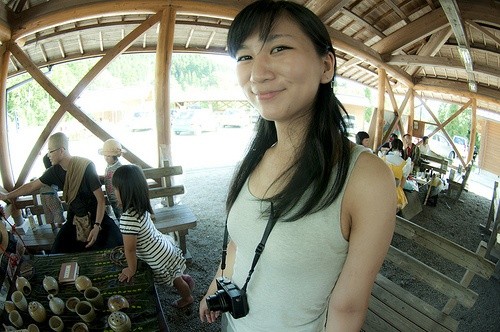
74;215;91;242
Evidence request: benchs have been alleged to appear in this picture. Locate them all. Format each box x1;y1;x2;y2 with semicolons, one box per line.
362;214;495;332
420;154;448;178
22;161;197;264
479;180;500;262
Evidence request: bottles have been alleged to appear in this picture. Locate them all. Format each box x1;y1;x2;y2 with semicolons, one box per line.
27;208;38;232
48;294;64;315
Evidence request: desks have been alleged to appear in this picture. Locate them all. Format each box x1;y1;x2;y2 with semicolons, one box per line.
1;252;168;332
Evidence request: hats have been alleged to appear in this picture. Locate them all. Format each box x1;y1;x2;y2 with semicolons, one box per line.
98;139;127;156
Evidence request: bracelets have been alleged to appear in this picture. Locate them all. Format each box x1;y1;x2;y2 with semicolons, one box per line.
93;223;102;231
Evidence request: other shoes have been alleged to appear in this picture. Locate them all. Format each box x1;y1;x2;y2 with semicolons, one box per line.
183;275;195;291
171;297;194;308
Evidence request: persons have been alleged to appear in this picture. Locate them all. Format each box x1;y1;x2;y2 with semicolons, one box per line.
112;165;195;307
97;139;123;221
40;154;65;230
199;0;397;332
356;132;429;214
0;132;124;254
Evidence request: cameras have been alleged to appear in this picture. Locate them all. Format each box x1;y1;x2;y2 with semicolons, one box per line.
206;276;249;320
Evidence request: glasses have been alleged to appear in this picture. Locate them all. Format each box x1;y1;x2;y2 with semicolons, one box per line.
48;146;65;153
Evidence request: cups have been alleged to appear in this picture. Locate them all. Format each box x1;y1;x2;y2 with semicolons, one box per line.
75;301;96;322
71;322;89;332
9;310;23;328
11;291;28;311
84;287;103;310
28;324;39;332
49;316;64;332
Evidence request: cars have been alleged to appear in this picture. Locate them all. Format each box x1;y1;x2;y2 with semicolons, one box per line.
125;105;244;135
413;129;456;161
341;114;369;137
453;135;468;158
